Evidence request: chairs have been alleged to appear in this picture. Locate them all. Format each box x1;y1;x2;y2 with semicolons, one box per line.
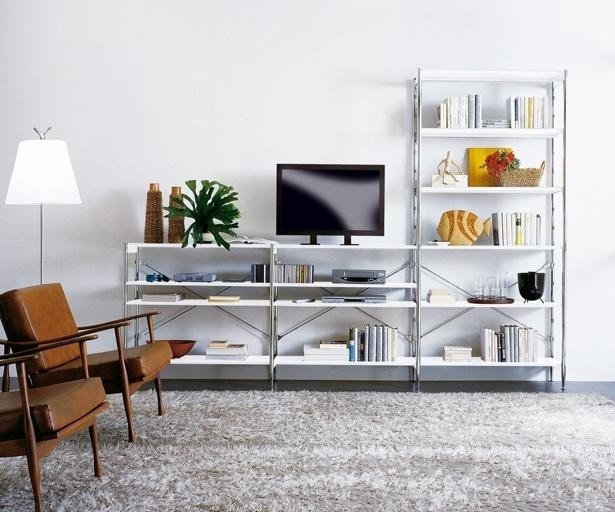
0;336;103;512
0;284;173;442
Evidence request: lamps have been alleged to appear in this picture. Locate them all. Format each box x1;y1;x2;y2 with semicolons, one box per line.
4;127;82;285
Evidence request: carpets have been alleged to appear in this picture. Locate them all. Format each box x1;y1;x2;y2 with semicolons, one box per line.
0;392;615;511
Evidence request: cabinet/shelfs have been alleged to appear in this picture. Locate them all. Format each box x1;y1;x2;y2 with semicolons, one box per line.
125;242;271;386
271;240;415;388
416;69;559;392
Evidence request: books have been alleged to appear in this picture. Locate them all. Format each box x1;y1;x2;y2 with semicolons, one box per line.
140;261;397;364
438;93;551;363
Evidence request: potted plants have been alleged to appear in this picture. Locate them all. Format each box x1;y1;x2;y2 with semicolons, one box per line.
166;179;239;250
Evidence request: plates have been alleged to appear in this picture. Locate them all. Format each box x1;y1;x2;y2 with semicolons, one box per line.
467;298;515;304
427;241;452;246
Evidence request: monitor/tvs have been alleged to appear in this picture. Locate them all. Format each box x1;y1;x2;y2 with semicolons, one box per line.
275;163;385;246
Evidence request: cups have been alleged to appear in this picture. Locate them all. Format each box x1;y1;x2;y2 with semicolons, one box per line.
474;269;510;300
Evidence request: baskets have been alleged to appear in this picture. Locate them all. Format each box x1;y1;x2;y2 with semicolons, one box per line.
499;160;546;187
